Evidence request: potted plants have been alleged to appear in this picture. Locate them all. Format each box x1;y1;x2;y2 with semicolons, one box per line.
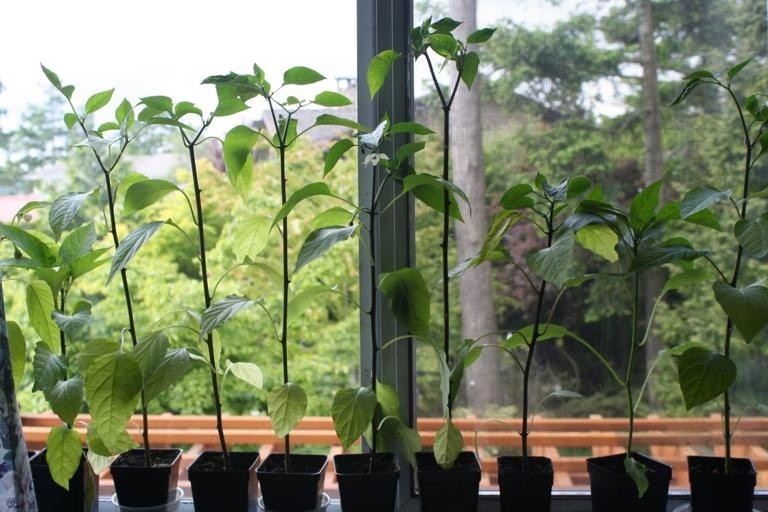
500;179;726;510
365;17;500;508
663;53;768;507
107;62;272;507
0;187;121;511
38;62;186;508
269;110;470;510
202;61;371;507
475;170;626;507
